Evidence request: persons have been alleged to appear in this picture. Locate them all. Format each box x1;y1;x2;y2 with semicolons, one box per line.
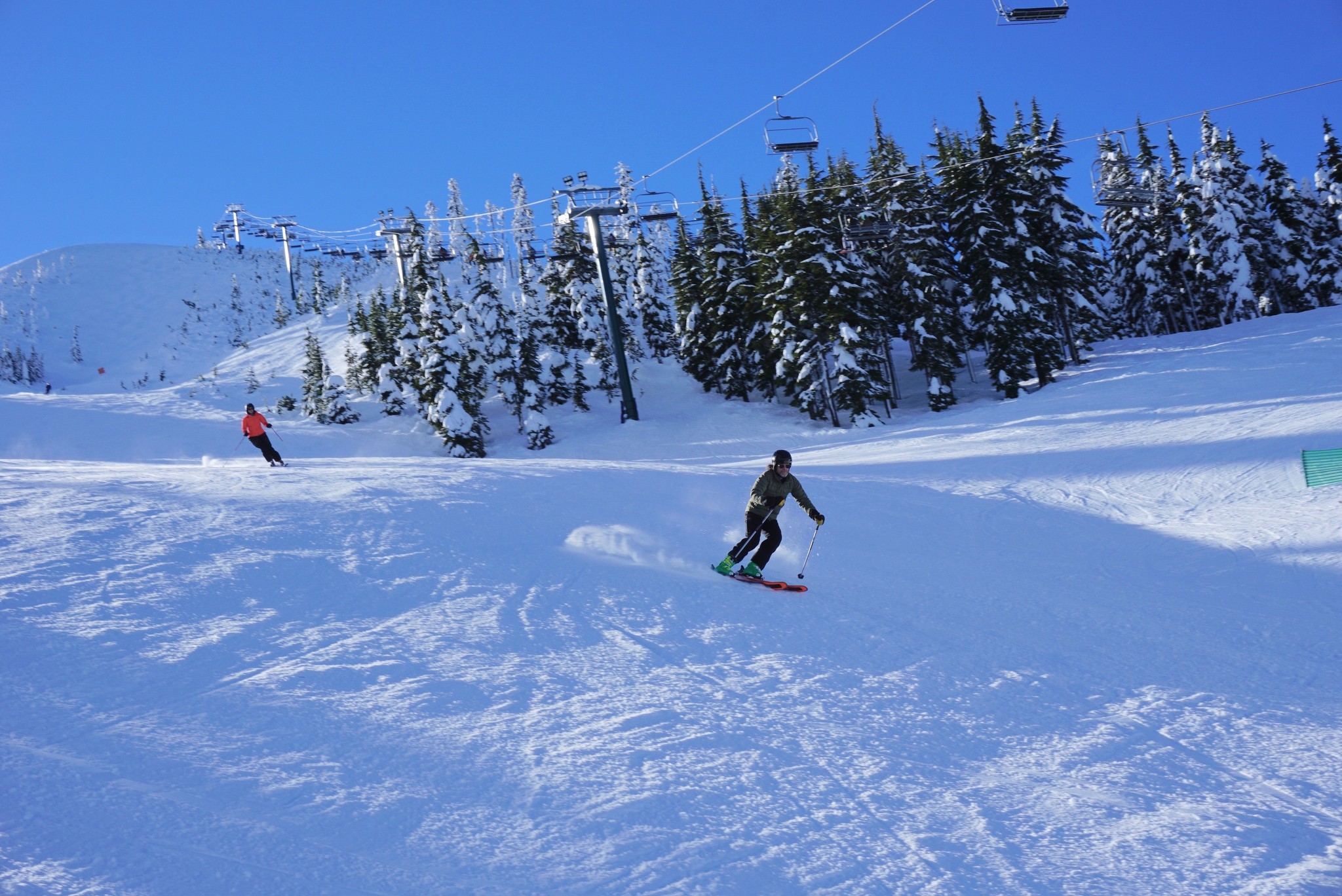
712;449;825;577
241;402;290;468
45;383;52;394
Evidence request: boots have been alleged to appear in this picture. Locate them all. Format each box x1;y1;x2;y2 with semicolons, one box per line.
742;560;765;580
715;555;735;575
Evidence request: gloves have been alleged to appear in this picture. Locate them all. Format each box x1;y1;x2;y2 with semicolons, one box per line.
266;423;272;428
815;514;825;525
762;496;773;506
244;431;249;436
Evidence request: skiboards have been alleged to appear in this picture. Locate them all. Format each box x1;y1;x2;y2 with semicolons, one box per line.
271;462;288;467
710;562;809;594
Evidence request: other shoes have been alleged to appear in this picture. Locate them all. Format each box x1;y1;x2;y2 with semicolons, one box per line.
271;462;275;466
278;460;284;465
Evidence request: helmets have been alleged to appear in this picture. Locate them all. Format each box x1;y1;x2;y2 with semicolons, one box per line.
245;403;254;410
772;450;792;464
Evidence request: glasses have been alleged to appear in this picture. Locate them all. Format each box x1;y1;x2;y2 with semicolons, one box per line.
249;408;254;410
777;464;791;468
